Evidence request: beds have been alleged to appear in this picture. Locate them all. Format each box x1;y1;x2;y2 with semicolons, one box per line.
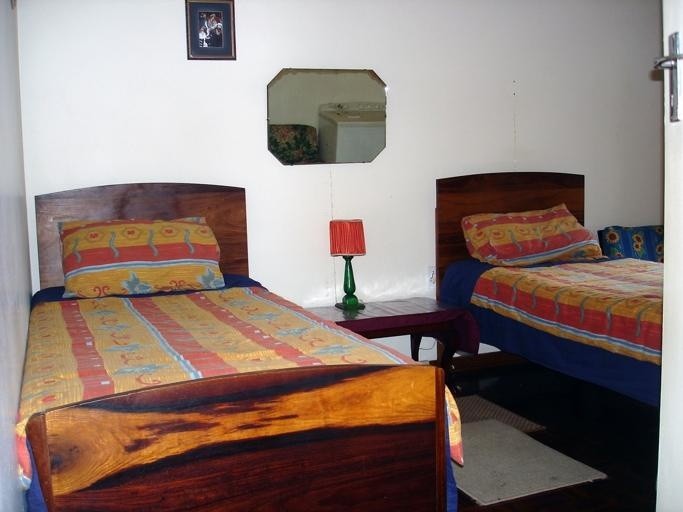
434;172;662;409
16;182;446;512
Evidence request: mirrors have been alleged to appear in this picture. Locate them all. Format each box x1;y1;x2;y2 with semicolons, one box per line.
266;67;387;165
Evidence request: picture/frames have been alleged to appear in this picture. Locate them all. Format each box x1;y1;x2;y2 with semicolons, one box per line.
184;0;236;60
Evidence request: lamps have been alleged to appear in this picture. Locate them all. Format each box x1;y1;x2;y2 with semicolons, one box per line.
328;219;367;312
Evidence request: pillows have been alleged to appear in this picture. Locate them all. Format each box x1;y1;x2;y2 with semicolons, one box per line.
460;202;601;267
53;214;226;300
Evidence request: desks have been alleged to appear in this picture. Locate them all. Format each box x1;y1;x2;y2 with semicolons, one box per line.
304;298;468;396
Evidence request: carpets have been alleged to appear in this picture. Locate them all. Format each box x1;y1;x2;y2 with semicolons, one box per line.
449;395;607;508
